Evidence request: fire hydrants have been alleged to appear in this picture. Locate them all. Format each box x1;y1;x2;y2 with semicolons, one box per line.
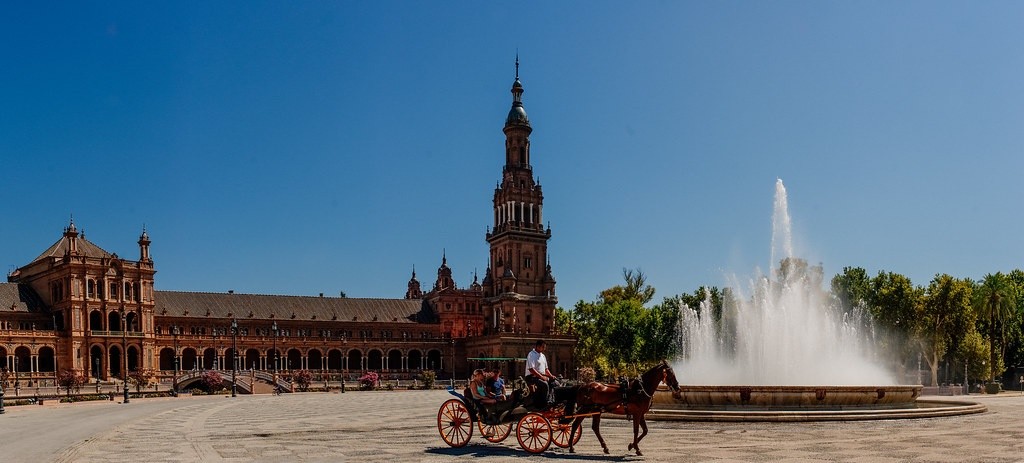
123;386;130;403
341;377;345;393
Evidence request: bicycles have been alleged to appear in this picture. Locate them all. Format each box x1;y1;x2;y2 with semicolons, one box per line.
272;388;283;396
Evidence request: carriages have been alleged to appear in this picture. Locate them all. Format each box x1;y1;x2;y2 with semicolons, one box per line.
437;359;681;456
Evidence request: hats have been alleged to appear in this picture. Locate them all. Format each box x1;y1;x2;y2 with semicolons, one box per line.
473;370;482;376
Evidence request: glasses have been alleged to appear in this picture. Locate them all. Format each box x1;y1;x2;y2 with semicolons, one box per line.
494;371;500;374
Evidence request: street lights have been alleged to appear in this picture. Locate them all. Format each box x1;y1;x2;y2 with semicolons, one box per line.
172;325;179;396
230;319;238;397
271;320;278;373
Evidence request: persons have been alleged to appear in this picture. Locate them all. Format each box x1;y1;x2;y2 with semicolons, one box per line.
470;368;506;404
524;340;559;399
273;374;282;392
